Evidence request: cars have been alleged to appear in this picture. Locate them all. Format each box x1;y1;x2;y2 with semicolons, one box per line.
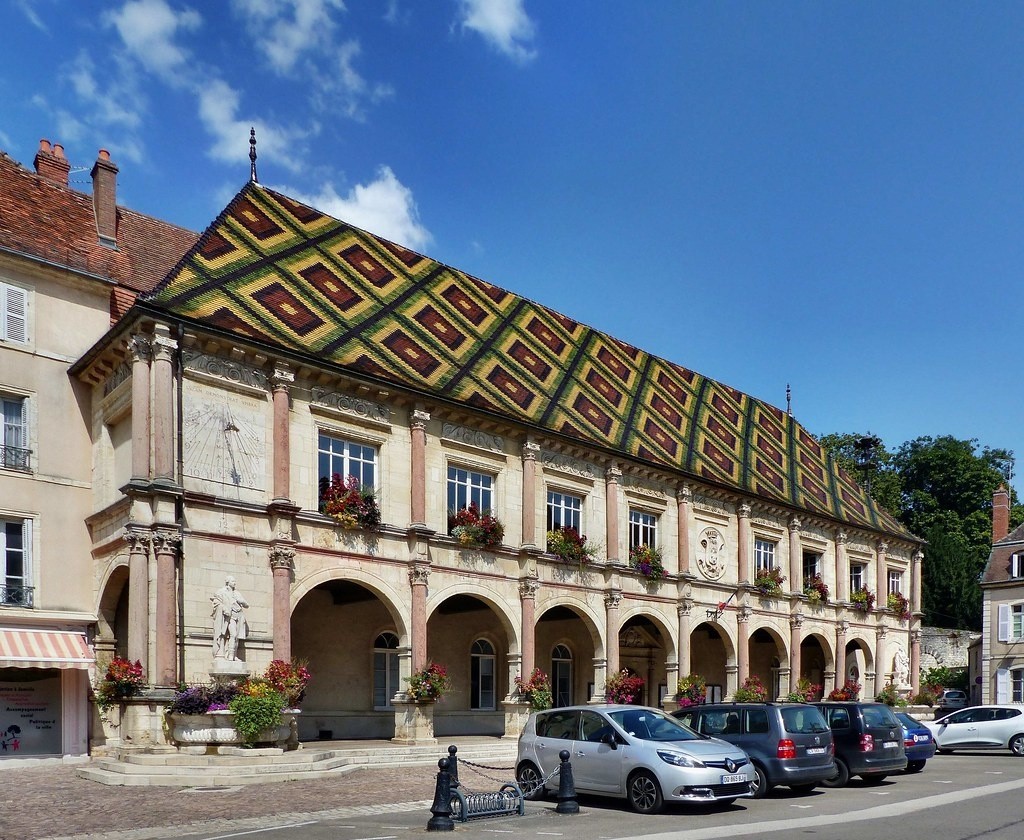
916;703;1024;758
939;689;969;713
828;712;936;774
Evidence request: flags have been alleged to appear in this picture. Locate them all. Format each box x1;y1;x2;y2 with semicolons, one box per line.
719;590;736;611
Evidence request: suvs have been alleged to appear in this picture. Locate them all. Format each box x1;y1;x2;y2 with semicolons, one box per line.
748;700;908;789
645;701;837;800
514;703;758;816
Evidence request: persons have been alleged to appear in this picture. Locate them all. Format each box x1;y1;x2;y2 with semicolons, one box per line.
209;574;250;661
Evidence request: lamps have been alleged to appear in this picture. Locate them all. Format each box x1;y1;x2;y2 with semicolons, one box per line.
947;628;959;638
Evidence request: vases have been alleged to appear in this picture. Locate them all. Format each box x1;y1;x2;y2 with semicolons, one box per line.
164;709;303;743
894;705;940;720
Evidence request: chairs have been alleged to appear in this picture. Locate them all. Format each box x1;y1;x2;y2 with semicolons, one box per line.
723;715;737;733
833;719;845;728
989;711;994;719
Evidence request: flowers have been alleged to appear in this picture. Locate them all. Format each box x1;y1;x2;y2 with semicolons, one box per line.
754;565;787;599
515;667;554;712
804;572;830;605
827;680;862;701
448;501;505;550
732;674;768;702
786;676;822;703
401;656;462;703
603;667;646;704
319;473;383;533
629;542;669;586
850;582;876;614
874;681;945;708
887;590;914;627
88;653;146;726
158;657;311;749
547;526;607;576
678;670;707;707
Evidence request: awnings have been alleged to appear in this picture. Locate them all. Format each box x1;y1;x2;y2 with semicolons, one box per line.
0;628;95;671
